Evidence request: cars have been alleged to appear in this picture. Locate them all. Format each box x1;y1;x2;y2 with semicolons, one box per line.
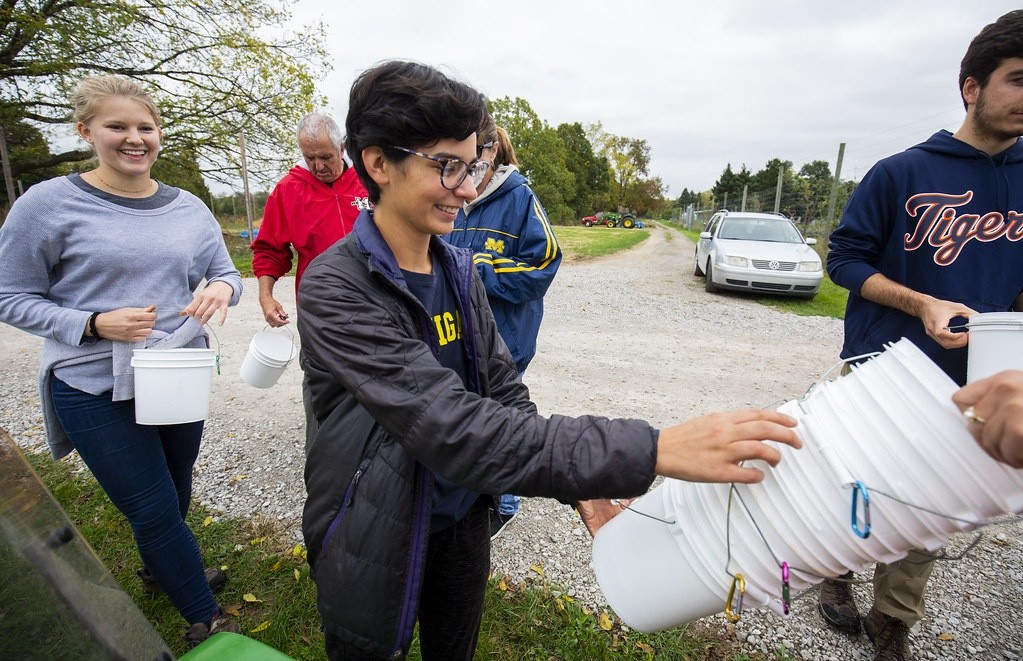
581;211;607;227
693;209;824;301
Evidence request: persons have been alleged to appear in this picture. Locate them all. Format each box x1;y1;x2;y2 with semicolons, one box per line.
297;61;801;659
819;7;1023;659
442;110;563;539
2;72;241;646
950;369;1022;470
252;114;378;329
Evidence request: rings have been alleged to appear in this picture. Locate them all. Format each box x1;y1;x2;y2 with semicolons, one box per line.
963;406;987;424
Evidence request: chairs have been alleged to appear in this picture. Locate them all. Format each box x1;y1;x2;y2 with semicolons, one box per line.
723;223;748;238
752;225;777;238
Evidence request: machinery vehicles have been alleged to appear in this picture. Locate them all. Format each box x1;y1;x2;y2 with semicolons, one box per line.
595;210;645;229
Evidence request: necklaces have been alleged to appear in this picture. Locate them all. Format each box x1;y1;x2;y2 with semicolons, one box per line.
93;170;153;193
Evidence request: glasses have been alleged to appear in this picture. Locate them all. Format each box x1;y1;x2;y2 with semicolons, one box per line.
387;144;490;191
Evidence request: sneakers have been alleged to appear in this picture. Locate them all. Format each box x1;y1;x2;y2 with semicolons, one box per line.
862;606;912;660
185;609;246;650
487;506;517;542
137;566;225;594
818;570;861;632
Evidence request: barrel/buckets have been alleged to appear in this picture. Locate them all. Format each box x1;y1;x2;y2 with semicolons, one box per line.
130;310;220;424
591;312;1023;634
240;322;296;388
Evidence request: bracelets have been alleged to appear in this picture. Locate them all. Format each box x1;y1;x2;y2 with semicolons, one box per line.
90;311;104;341
